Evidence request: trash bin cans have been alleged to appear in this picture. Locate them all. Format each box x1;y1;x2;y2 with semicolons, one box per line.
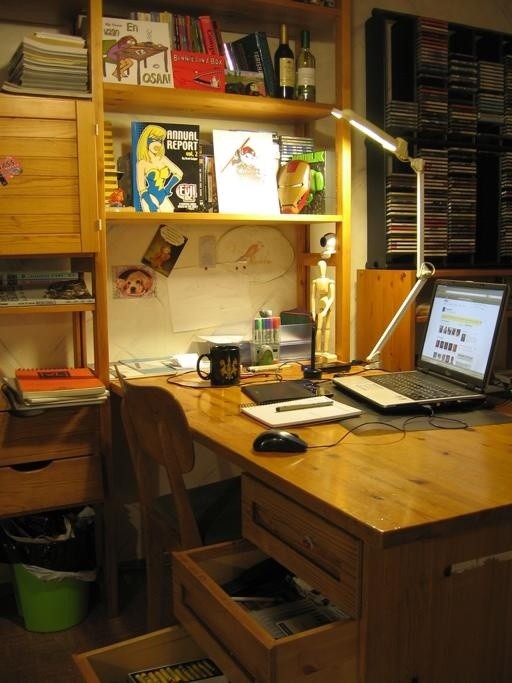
1;504;96;633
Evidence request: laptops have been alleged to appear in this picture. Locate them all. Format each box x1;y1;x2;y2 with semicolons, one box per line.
334;277;508;414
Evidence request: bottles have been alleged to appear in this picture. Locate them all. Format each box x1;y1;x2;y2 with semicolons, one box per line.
296;29;315;100
274;23;296;98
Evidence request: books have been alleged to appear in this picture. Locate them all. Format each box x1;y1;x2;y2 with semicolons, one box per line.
114;356;208;379
2;263;96;310
386;15;512;275
131;122;200;215
2;367;112;416
127;8;223;58
230;31;280;102
276;136;318;172
237;396;362;428
1;32;92;100
198;153;218;213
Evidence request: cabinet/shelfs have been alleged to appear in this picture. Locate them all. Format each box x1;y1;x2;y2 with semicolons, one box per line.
363;7;511;362
0;0;349;626
72;346;512;683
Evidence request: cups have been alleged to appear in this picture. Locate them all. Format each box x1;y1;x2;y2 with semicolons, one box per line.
196;345;241;387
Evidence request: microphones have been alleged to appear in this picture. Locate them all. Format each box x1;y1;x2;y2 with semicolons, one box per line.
304;325;323;378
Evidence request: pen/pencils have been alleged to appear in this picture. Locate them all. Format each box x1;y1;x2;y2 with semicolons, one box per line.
255;318;280;345
230;596;284;601
250;598;334;640
247;363;293;372
276;401;333;412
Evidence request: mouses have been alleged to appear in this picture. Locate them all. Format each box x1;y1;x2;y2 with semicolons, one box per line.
255;431;306;452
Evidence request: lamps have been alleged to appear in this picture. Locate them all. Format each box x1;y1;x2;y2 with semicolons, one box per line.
328;106;435;371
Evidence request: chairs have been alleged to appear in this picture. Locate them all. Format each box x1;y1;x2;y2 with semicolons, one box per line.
115;367;241;632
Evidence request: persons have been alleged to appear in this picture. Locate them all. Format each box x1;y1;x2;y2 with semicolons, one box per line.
136;125;183;212
107;35;136;79
